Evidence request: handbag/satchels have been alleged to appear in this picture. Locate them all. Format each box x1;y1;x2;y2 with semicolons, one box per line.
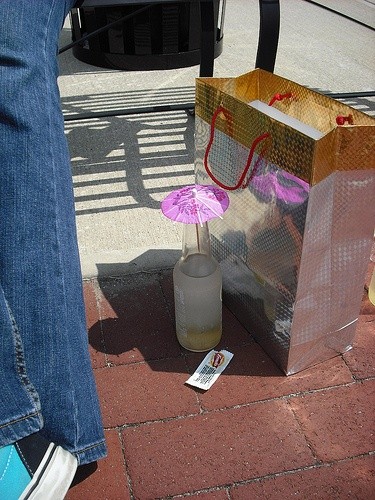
191;67;375;376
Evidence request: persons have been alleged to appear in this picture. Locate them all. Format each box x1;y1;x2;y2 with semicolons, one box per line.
0;0;111;499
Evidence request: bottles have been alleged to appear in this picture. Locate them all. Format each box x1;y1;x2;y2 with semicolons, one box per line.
172;221;223;352
264;281;281;322
368;235;375;306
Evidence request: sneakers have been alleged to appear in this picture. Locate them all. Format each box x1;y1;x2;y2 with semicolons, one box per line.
0;432;79;500
